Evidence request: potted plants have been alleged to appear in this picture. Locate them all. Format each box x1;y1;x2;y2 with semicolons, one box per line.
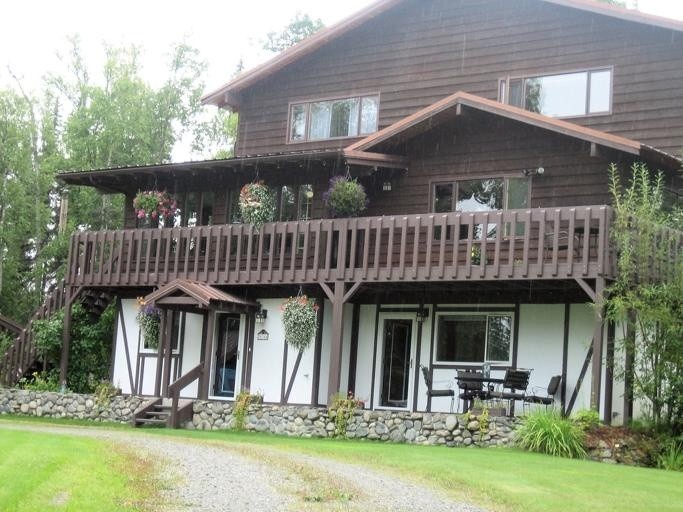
93;381;122;412
231;391;264;431
324;391;366;439
458;396;506;438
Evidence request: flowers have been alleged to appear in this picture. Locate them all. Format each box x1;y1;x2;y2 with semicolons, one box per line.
135;296;164;348
238;182;276;228
134;192;177;220
281;295;319;349
321;173;370;218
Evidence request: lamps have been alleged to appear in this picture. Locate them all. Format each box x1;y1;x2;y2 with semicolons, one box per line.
521;164;544;178
382;173;391;192
254;308;267;323
416;308;429;321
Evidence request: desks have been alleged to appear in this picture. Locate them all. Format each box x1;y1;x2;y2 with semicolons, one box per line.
454;376;504;414
573;227;600;261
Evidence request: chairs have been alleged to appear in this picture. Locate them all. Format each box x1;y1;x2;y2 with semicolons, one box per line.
421;366;455;413
456;368;487;415
491;368;534;416
522;375;561;416
536;206;579;263
